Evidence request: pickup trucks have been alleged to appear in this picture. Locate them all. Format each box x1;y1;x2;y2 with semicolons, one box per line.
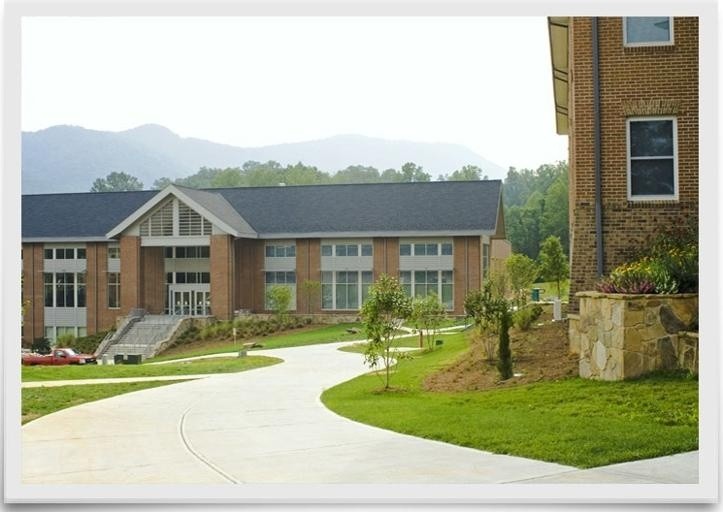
22;347;96;366
